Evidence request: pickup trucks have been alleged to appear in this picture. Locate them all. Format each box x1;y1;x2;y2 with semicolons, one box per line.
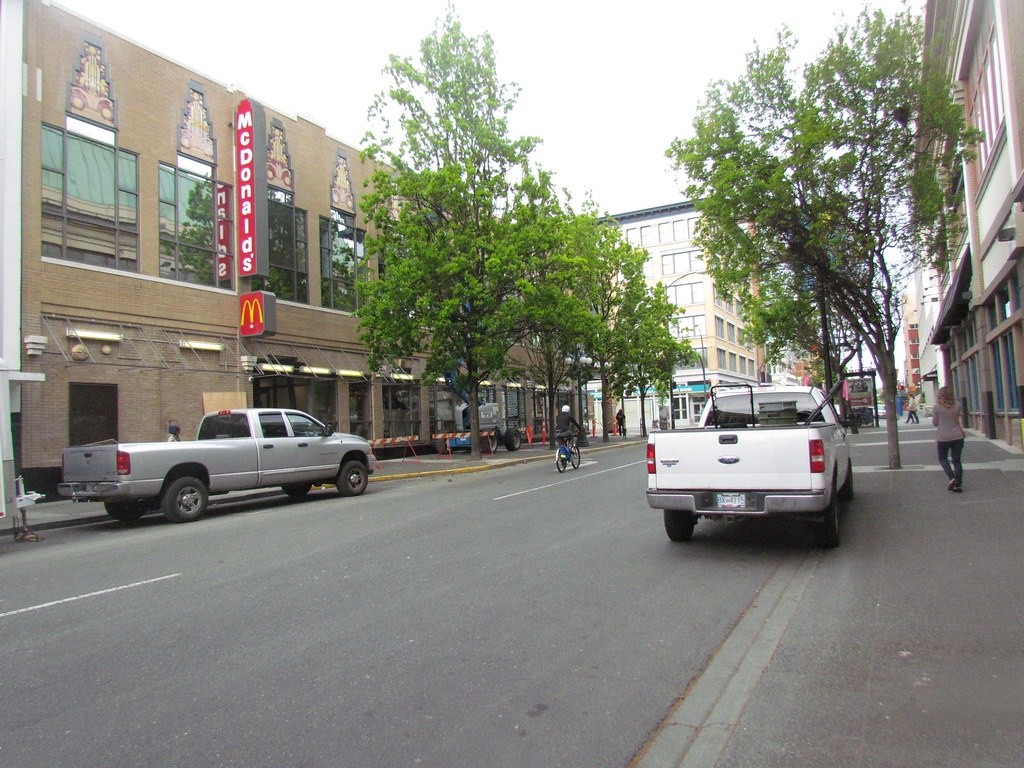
644;383;856;549
55;406;380;524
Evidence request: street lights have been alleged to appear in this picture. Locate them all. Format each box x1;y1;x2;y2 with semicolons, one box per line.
663;268;709;429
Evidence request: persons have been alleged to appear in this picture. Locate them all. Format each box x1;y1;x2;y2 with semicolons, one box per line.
931;386;966;493
616;409;626;436
850;382;868;393
556;405;582;466
904;394;919;424
168;426;181;442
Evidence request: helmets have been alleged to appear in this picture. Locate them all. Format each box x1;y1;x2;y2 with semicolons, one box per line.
562;405;570;413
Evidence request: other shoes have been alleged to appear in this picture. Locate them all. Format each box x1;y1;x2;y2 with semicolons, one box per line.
953;486;961;492
905;422;908;423
911;422;914;423
915;421;919;423
570;449;576;454
947;479;955;491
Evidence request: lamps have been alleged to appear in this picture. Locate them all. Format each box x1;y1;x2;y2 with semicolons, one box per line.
998;228;1014;241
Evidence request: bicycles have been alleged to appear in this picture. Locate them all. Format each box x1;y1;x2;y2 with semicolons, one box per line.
555;428;585;473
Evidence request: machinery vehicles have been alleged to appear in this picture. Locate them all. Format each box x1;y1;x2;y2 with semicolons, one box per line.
432;400;523;455
